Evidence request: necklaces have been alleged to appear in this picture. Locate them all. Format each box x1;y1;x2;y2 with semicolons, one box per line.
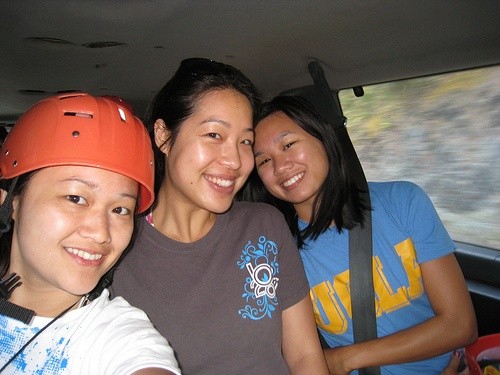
0;294;86;374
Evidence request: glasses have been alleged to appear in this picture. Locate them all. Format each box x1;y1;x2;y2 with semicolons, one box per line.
175;55;258;95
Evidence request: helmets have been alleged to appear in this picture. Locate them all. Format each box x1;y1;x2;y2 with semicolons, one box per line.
0;92;155;215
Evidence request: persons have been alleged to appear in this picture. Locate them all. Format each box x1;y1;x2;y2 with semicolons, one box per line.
237;96;477;375
102;58;332;375
0;90;183;375
